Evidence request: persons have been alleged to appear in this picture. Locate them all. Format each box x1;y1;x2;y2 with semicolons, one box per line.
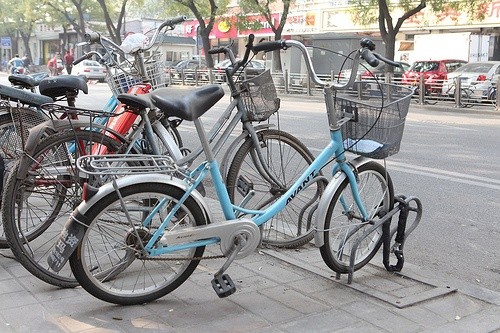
64;50;74;74
9;54;24;74
48;58;56;75
22;54;31;69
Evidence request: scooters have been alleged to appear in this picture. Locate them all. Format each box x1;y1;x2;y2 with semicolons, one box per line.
8;59;24;75
56;59;64;73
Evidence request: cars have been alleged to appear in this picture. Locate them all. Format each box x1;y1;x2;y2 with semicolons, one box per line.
401;58;468;97
442;60;500;103
172;59;267;75
339;61;412;100
61;59;107;83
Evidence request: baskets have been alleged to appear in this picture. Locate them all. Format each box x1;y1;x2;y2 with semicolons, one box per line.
226;68;280;121
323;81;414;158
103;61;166;105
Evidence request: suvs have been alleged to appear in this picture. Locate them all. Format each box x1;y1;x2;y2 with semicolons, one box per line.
110;59;157;76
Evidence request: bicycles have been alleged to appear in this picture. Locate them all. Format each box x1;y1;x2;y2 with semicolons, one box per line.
0;33;328;289
46;38;416;306
0;50;183;158
424;73;497;109
0;14;206;250
